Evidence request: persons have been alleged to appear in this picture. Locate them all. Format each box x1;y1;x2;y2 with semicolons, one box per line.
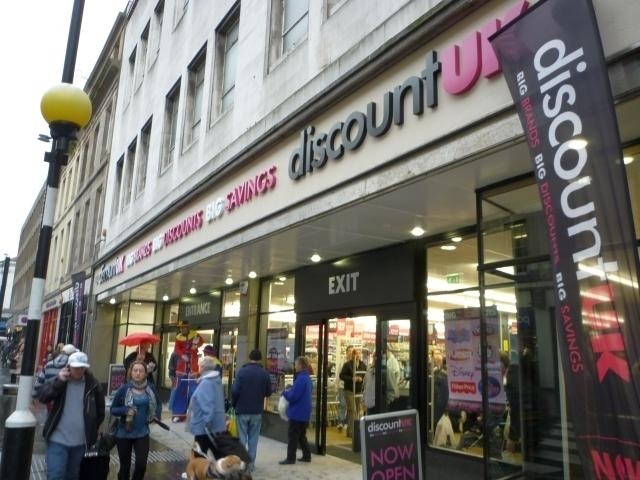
336;346;520;459
0;334;105;479
111;359;161;479
181;356;227;478
168;319;205;421
277;357;314;465
203;343;222;382
122;340;158;386
229;350;272;472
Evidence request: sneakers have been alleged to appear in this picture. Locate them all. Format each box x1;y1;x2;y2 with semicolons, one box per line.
337;423;343;432
298;458;311;462
279;460;295;464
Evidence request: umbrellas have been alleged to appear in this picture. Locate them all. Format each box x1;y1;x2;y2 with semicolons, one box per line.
119;332;160;354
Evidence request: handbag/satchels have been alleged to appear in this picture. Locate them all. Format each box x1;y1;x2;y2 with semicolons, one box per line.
79;454;109;480
225;413;238;438
100;418;118;452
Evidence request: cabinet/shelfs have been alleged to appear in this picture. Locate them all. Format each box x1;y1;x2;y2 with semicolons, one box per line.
221;335;443;428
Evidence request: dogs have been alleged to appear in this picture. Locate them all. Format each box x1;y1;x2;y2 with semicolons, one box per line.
185;448;247;480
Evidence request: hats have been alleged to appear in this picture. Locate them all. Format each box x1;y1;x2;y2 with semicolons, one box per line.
62;344;80;354
68;351;90;368
204;345;214;353
249;351;261;360
